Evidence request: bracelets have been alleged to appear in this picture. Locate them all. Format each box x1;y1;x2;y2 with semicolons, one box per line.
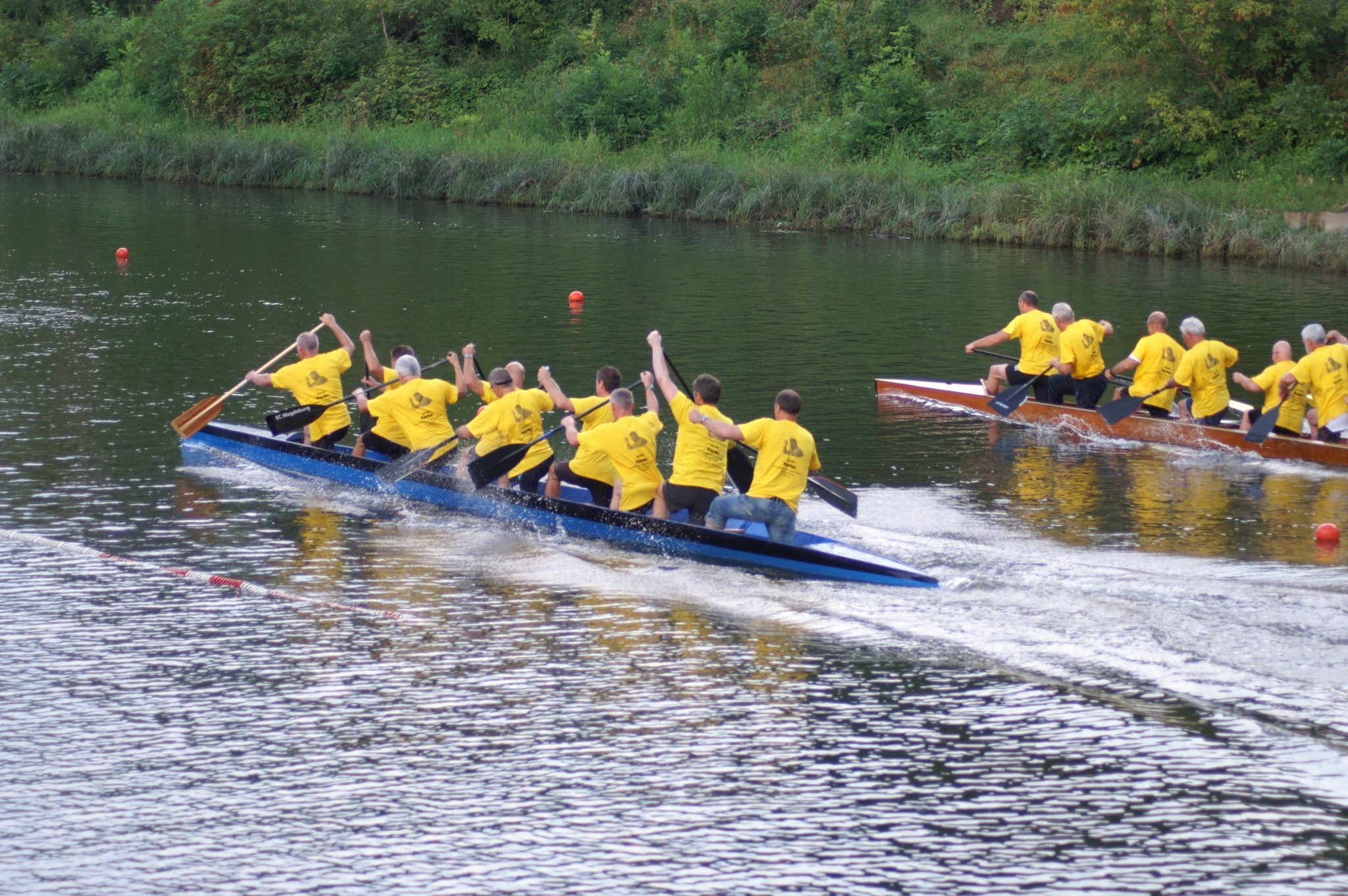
354;391;365;397
1110;369;1116;377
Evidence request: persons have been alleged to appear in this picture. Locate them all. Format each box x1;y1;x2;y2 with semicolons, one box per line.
1104;311;1188;419
1047;302;1113;411
688;389;821;546
965;291;1060;404
1278;323;1348;444
352;329;664;513
1232;341;1315;438
244;313;355;451
1165;316;1239;427
646;330;737;528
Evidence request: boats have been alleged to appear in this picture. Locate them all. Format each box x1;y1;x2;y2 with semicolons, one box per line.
875;377;1348;471
184;423;941;591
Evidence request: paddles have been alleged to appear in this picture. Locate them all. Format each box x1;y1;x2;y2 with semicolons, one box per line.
374;384;543;490
471;344;487;380
264;352;451;438
662;346;754;495
466;380;642;492
170;322;325;440
1116;374;1255;413
735;439;858;519
986;365;1053;419
1095;386;1169;426
972;348;1133;386
359;358;373;435
1243;339;1330;444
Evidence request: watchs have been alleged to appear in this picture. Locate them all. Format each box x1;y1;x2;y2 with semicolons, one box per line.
464;354;474;358
700;415;708;424
644;384;654;390
